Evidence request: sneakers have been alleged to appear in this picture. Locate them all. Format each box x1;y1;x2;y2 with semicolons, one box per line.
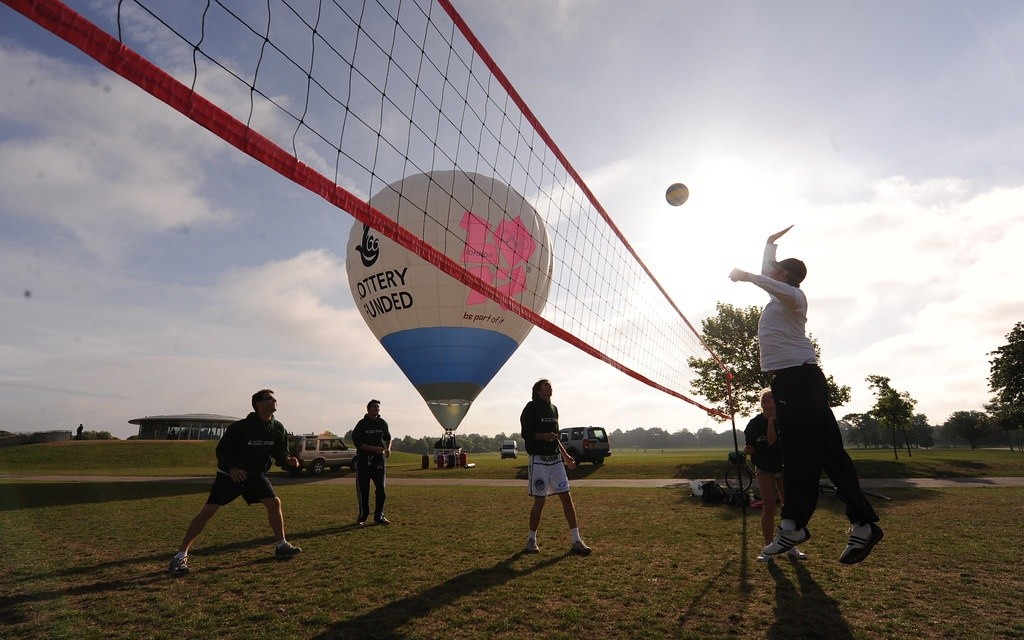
571;540;592;554
275;542;302;559
526;537;540;553
169;555;189;574
838;521;883;565
786;546;807;559
757;549;774;562
763;524;810;555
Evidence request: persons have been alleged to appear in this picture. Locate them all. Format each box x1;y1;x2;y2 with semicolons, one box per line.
520;380;592;553
351;400;392;526
744;388;806;562
77;424;83;440
728;225;883;565
169;390;301;575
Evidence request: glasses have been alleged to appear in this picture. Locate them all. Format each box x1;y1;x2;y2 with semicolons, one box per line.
263;397;276;401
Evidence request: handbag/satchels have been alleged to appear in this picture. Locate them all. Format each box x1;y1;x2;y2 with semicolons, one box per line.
690;480;704;496
727;488;747;507
701;481;725;504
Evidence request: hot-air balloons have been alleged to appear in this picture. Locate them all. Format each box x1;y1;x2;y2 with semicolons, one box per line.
346;171;554;464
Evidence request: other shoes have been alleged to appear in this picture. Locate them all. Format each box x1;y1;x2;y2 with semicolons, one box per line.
356;519;365;527
374;514;390;525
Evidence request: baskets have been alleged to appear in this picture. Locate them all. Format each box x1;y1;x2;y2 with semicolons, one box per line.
728;451;747;466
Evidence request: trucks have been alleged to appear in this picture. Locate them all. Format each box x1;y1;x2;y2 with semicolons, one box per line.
499;441;518;459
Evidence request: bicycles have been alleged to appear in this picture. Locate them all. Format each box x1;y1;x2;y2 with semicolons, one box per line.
725;450;756;493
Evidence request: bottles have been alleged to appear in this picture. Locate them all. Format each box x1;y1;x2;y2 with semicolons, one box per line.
749;489;754;502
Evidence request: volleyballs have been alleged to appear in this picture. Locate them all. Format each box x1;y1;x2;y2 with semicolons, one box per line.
666;183;689;206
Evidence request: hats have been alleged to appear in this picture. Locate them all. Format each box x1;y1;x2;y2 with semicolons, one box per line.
769;258;806;284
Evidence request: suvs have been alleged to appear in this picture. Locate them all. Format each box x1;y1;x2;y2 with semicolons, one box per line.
274;434;358;479
559;426;612;467
